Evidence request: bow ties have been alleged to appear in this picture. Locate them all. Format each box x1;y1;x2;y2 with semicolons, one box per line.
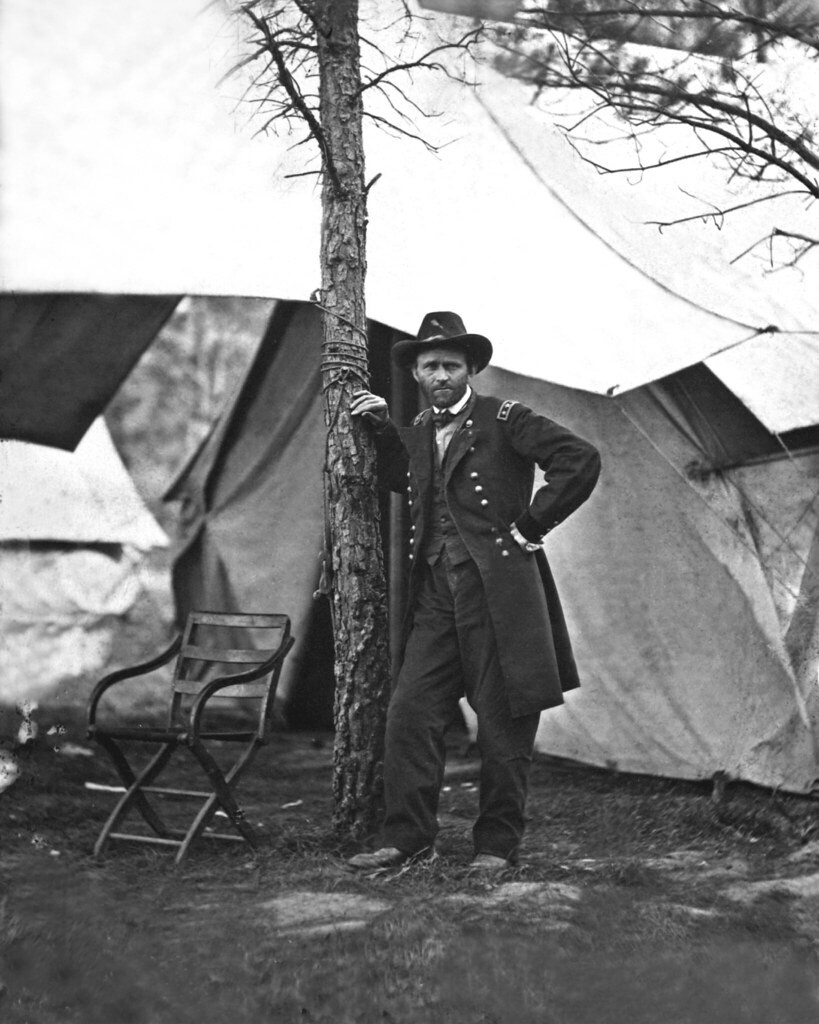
431;412;458;429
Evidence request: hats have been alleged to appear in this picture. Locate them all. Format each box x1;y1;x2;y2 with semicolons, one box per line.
391;311;493;375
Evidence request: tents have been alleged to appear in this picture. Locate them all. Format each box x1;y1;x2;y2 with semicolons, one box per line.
0;0;819;791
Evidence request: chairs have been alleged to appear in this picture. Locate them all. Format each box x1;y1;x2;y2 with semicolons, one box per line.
86;610;295;869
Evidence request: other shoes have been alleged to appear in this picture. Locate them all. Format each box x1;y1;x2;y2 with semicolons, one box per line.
347;848;417;867
469;852;511;872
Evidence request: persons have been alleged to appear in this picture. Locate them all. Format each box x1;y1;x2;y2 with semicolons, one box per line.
348;312;601;883
